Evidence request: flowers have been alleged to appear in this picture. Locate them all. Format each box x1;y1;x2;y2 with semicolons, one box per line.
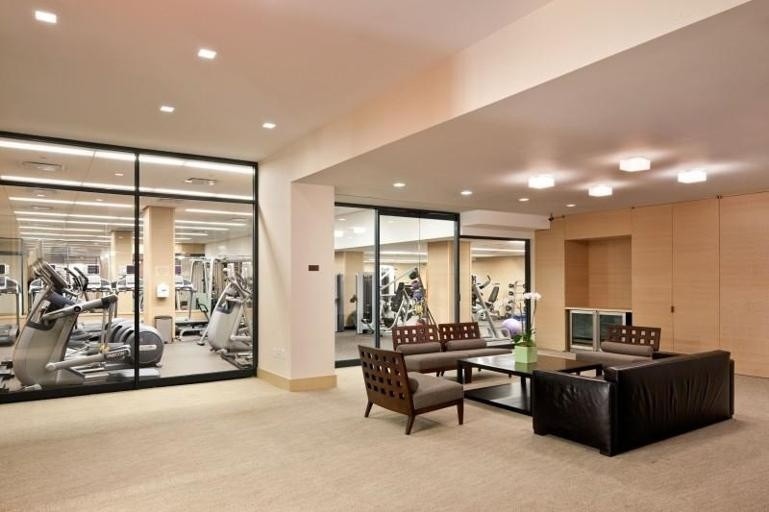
489;277;544;347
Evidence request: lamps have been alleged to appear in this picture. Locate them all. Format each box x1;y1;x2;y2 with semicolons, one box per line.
676;167;708;185
617;156;652;172
527;174;556;189
587;184;616;198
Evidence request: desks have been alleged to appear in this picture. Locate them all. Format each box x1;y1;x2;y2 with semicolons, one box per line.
452;349;604;421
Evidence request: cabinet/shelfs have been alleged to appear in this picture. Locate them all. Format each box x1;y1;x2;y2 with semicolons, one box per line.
632;199;720;364
721;189;769;378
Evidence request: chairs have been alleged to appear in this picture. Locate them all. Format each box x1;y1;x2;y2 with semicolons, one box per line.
573;322;664;371
357;343;468;437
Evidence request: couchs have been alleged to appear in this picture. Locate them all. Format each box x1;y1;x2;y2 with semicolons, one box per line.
525;348;740;459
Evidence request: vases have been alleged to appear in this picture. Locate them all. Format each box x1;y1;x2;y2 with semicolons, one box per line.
512;345;541;366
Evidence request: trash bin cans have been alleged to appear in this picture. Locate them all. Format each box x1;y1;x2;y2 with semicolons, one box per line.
154;316;172;344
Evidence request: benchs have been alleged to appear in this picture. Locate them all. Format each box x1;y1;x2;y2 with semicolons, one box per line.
391;321;516;385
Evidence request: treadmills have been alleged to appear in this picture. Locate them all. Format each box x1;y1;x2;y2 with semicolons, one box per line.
0;274;22;346
13;241;163;388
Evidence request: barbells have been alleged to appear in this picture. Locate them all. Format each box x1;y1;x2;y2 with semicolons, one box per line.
504;281;525;321
